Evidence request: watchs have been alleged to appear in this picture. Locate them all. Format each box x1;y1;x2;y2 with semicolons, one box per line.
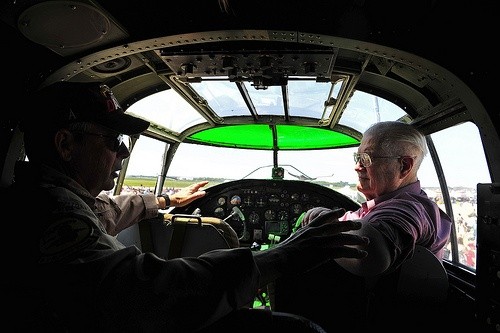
157;193;171;210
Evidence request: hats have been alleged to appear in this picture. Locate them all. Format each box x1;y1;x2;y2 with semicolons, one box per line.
23;82;150;141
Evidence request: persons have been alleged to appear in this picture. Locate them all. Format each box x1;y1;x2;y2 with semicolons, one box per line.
302;120;453;276
164;186;175;195
457;208;477;267
0;82;369;333
127;184;150;194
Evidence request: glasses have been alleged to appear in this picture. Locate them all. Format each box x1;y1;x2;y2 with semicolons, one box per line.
353;152;417;167
50;129;123;151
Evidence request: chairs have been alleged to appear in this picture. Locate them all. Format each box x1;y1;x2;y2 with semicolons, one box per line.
141;214;238;262
372;246;450;333
